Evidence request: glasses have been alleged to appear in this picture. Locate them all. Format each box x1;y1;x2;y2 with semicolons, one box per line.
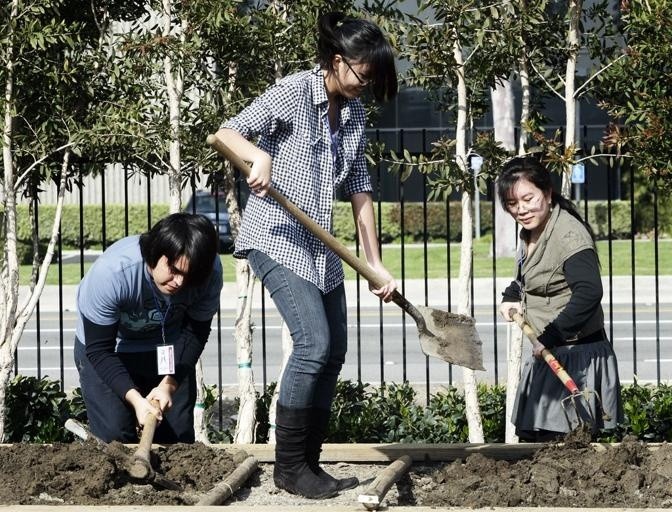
504;190;545;214
339;53;376;90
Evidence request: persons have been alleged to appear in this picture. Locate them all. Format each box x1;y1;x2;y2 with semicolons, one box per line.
73;213;223;443
499;157;619;442
215;11;398;497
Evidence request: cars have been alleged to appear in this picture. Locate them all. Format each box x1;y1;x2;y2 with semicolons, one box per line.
184;190;235;253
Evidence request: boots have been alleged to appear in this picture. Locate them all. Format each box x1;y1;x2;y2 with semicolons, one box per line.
273;401;359;500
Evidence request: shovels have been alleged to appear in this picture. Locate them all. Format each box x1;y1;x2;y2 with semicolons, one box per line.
207;136;487;369
